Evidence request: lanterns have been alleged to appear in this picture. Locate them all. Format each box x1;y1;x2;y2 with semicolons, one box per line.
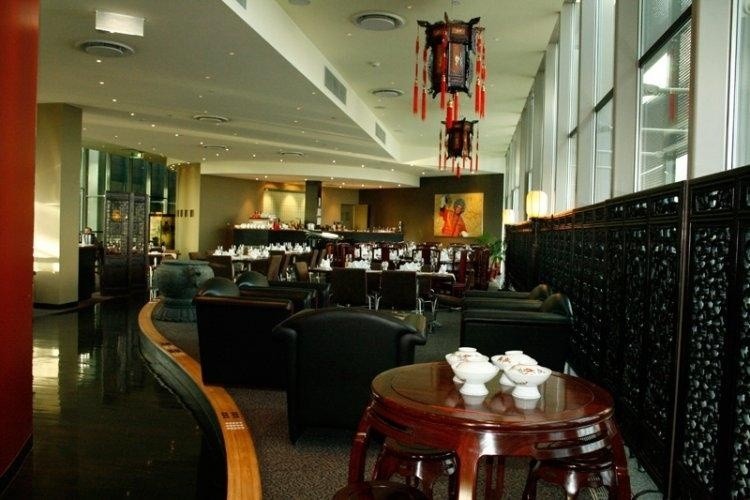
438;113;480;181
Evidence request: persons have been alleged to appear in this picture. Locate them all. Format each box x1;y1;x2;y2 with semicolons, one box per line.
79;226;98;245
437;195;468;237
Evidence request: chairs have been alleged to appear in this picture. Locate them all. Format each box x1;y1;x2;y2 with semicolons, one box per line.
190;240;574;431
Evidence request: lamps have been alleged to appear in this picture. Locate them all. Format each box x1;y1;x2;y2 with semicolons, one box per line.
194;115;228;126
526;190;548;245
503;210;516;227
351;10;405;32
79;39;134;59
373;88;403;99
441;92;480;179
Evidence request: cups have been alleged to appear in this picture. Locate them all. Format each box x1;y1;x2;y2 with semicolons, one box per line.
318;258;448;275
215;241;311;259
240;221;289;230
353;240;471;261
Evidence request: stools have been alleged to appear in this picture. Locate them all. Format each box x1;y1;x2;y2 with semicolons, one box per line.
331;479;427;499
377;432;460;500
524;449;616;500
